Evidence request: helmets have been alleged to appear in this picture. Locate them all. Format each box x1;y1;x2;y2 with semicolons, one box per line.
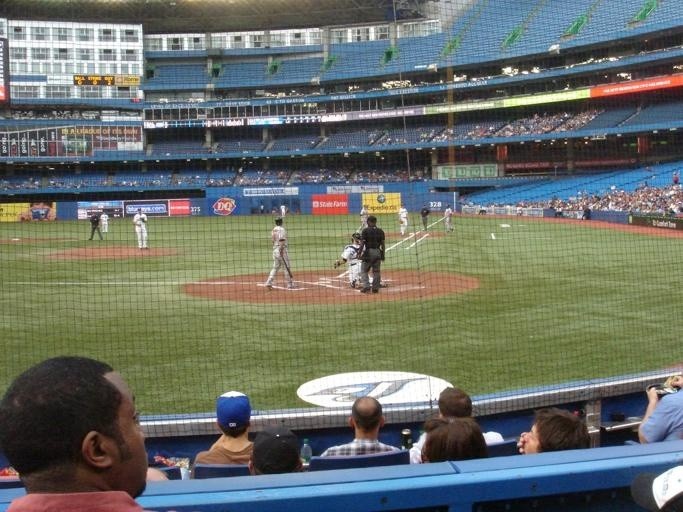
352;232;362;240
274;216;283;221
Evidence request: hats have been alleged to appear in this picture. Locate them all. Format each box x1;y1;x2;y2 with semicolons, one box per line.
215;390;250;429
252;426;302;474
366;215;378;224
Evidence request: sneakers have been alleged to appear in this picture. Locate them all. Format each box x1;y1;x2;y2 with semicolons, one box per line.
264;285;273;292
287;285;297;289
351;282;389;293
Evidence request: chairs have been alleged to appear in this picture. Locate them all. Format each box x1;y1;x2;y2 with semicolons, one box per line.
146;124;442;153
0;423;249;489
1;167;433;193
442;1;683;220
590;422;642;447
484;438;518;457
309;450;409;472
142;33;439;109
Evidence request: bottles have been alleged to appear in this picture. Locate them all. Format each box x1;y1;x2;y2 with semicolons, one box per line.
401;429;414;451
301;439;312;465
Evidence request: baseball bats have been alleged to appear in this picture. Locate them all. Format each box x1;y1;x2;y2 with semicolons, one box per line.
281;256;293;278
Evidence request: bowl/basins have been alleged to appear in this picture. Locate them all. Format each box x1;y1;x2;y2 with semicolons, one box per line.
646;383;678;397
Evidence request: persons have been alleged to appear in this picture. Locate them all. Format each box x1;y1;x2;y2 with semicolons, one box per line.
468;172;683;214
188;390;255;481
248;423;303;476
637;375;683;444
408;418;449;464
417;387;504;445
386;108;597;145
0;355;147;512
318;395;401;458
516;407;590;454
423;416;488;464
147;466;168;482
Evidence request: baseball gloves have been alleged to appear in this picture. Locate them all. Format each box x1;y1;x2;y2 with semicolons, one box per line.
334;259;341;268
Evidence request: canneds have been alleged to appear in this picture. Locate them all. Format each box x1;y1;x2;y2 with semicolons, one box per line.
402;428;412;448
